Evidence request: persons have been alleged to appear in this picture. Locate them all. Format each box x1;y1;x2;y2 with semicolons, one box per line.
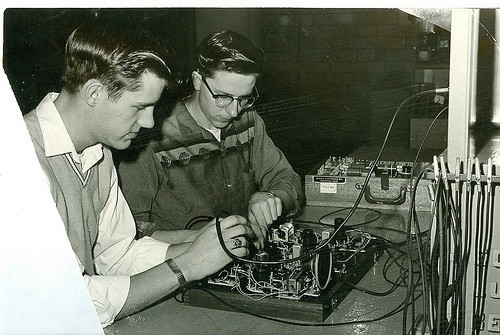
25;19;253;329
117;29;304;250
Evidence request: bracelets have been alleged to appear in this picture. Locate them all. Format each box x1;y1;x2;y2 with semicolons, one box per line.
248;191;275;202
164;258;187;288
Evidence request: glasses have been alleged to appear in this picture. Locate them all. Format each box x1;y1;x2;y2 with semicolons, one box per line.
198;72;259;108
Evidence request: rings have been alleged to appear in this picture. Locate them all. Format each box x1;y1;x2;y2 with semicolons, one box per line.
233;237;242;248
